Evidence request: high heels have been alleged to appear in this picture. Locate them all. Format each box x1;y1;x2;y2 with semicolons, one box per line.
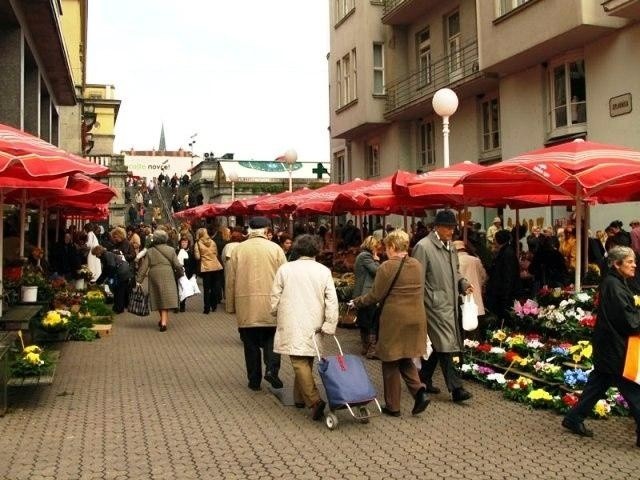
210;305;217;312
203;308;210;315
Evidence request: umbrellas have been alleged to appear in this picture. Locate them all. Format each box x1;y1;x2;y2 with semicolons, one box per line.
0;122;118;221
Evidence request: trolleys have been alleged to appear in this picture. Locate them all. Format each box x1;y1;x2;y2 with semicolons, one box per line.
309;331;382;430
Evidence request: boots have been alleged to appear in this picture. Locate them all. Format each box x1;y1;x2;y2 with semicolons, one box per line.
367;334;380;360
361;334;367;355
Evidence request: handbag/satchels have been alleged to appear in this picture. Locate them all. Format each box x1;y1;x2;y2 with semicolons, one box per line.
173;265;184;280
622;336;639;385
127;289;150;316
356;303;379;330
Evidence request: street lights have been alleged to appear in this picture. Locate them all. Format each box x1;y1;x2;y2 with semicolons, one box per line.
228;171;237;201
284;150;297;192
431;90;460;170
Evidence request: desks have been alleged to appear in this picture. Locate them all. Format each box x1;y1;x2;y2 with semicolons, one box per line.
1;330;60;419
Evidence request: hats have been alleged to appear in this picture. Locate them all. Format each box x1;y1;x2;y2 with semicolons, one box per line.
427;210;458;227
629;218;640;226
249;217;272;230
491;217;501;223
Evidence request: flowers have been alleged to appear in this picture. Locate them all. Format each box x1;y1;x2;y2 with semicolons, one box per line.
448;282;632;420
4;246;117;343
8;343;56;379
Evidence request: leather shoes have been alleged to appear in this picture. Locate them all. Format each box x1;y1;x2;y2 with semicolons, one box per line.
453;388;474;403
412;393;430;415
379;404;400;417
160;326;166;332
313;399;326;421
248;382;261;391
264;370;283;389
425;384;440;394
561;419;596;437
296;401;306;408
159;321;162;327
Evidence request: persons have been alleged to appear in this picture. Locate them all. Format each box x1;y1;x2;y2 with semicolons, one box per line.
3;164;639;421
560;244;640;450
